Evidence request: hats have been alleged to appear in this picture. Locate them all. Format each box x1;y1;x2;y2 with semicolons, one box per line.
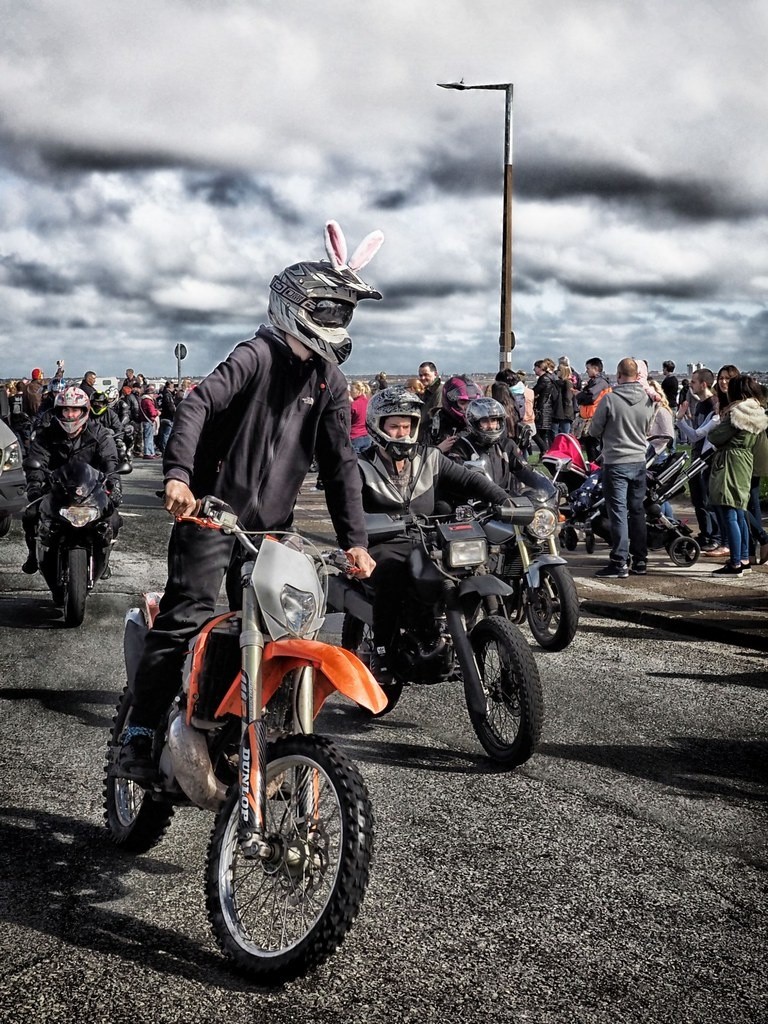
133;382;143;388
142;388;155;395
122;386;132;395
32;369;40;380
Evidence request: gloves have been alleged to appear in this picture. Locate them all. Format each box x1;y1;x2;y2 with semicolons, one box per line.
548;477;568;497
26;482;43;503
115;438;125;449
107;479;122;508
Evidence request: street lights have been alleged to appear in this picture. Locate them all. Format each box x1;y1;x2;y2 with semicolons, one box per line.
435;76;515;373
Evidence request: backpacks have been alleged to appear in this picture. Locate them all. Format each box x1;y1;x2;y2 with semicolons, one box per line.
509;406;533;451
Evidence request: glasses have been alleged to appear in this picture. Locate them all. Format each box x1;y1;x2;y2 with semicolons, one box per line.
366;423;419;462
307;299;353;329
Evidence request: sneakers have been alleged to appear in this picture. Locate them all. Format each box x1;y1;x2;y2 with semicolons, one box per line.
632;561;647;574
741;561;752;573
712;564;743;578
595;564;629;577
118;725;158;775
368;639;400;685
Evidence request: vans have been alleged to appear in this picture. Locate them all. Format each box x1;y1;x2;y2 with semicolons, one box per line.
171;379;191;391
75;376;120;393
147;378;168;391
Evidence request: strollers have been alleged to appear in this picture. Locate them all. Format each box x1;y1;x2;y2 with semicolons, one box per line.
559;435;716;568
542;432;603;492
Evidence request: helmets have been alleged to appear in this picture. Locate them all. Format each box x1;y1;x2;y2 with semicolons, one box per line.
466;397;506;443
441;377;485;423
106;388;119;409
49;378;66;395
366;388;425;455
53;386;90;435
87;390;109;418
268;262;381;366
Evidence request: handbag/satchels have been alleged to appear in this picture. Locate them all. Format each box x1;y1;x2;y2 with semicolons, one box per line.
152;416;160;435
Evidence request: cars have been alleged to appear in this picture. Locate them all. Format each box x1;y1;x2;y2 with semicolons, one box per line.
0;418;28;538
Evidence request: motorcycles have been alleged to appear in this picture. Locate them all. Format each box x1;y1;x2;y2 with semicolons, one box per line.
99;495;391;986
21;457;133;628
311;496;546;764
463;457;580;652
17;400;134;448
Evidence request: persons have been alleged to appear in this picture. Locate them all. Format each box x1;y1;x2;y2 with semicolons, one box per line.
114;219;381;783
0;357;768;687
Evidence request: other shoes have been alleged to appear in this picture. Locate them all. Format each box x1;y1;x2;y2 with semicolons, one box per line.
100;566;110;580
132;449;159;460
20;556;39;574
749;556;757;565
705;546;730;556
701;541;719;551
759;544;768;565
